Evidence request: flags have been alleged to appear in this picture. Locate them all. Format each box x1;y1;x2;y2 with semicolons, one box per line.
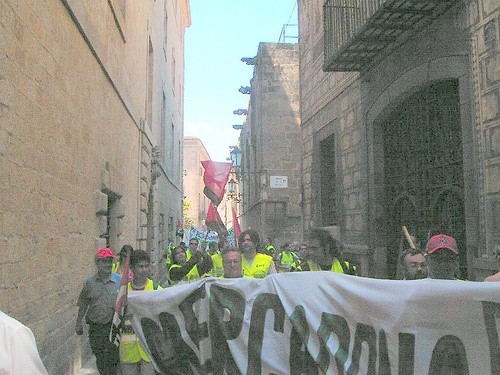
200;161;241;236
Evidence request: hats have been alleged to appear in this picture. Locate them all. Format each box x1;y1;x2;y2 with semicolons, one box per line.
426;234;459;254
96;248;117;258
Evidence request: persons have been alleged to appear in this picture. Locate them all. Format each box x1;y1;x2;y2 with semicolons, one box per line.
75;230;500;375
0;310;49;375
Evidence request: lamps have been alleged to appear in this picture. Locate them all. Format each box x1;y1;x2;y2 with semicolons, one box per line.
228;179;243;202
